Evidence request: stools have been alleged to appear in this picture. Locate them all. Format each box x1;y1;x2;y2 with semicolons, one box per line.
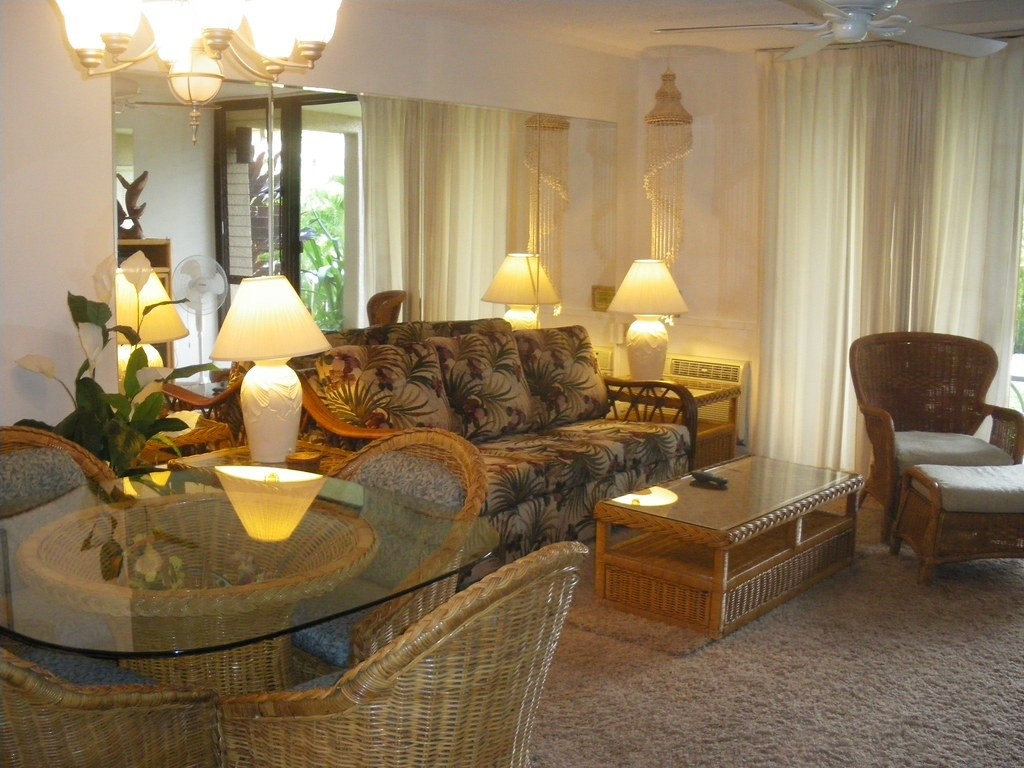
888;463;1024;585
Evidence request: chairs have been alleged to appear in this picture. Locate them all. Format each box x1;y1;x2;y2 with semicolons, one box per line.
0;425;591;768
847;331;1024;545
366;290;407;324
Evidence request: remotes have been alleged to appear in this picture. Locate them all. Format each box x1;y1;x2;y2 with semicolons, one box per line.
691;471;728;485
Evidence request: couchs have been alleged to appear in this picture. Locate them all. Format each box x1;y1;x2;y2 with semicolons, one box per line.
160;316;512;452
295;325;699;592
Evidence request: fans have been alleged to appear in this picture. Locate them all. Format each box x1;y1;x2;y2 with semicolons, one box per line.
171;254;228;385
650;1;1008;62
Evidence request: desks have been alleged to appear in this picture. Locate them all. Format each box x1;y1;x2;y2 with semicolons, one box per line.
0;467;502;697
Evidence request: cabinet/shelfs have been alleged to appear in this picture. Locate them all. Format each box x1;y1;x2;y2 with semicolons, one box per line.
117;238;175;386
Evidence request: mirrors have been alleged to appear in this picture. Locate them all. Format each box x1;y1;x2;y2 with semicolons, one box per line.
109;68;619;391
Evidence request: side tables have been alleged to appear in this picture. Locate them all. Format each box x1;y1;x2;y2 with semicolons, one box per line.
168;436;354;494
604;374;741;473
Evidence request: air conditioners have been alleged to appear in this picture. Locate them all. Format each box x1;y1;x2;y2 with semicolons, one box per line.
670;354;747;447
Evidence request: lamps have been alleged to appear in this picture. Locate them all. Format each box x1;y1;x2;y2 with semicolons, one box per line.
210;275;333;464
117;268;191;397
606;259;689;395
585;120;616;266
640;33;694;268
523;114;571;318
214;465;330;542
54;1;342;146
481;254;560;332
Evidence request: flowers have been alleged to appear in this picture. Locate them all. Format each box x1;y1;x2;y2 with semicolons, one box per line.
12;253;189;478
80;476;200;591
116;251;222;400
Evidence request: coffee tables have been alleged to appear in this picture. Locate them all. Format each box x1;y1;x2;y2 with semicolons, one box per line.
591;454;867;643
